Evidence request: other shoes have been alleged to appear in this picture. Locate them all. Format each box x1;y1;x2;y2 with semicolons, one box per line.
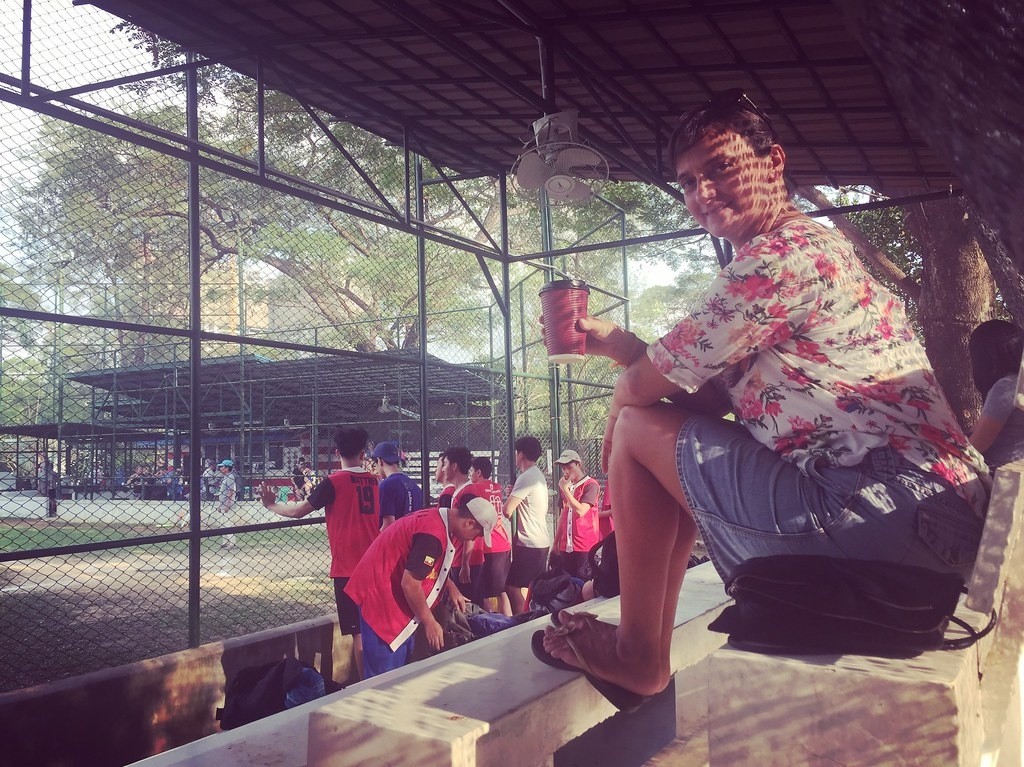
216;543;237;549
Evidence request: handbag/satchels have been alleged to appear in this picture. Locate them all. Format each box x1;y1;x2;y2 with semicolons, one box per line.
706;555;998;655
220;657;345;731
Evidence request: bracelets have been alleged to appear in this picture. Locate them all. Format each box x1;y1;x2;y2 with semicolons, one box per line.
609;332;638;369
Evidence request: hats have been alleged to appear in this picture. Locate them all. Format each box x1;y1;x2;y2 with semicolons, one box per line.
217;460;234;466
554;450;582;465
373;442;406;465
466;496;498;548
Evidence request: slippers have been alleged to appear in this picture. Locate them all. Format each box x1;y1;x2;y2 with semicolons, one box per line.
531;622;642;715
551;610;655;701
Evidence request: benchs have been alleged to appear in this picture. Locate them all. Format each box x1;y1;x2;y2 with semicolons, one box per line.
707;452;1024;767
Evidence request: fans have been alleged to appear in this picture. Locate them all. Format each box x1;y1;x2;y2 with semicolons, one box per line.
377;396;394;414
510;110;610;207
204;423;218;435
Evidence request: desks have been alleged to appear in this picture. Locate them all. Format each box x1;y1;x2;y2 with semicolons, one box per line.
306;560;736;767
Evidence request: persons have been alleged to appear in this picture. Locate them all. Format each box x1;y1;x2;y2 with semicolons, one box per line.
127;461;217;501
257;425;382;681
530;91;994;716
597;476;616;539
362;440;513;619
343;497;498;677
502;436;552;616
549;449;601;581
215;458;238;550
969;320;1023;476
289;456;317;500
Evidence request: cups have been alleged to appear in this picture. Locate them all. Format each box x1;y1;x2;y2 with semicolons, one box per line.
538;279;590;364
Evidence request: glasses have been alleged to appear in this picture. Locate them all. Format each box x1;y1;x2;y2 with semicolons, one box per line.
710;87;780;141
